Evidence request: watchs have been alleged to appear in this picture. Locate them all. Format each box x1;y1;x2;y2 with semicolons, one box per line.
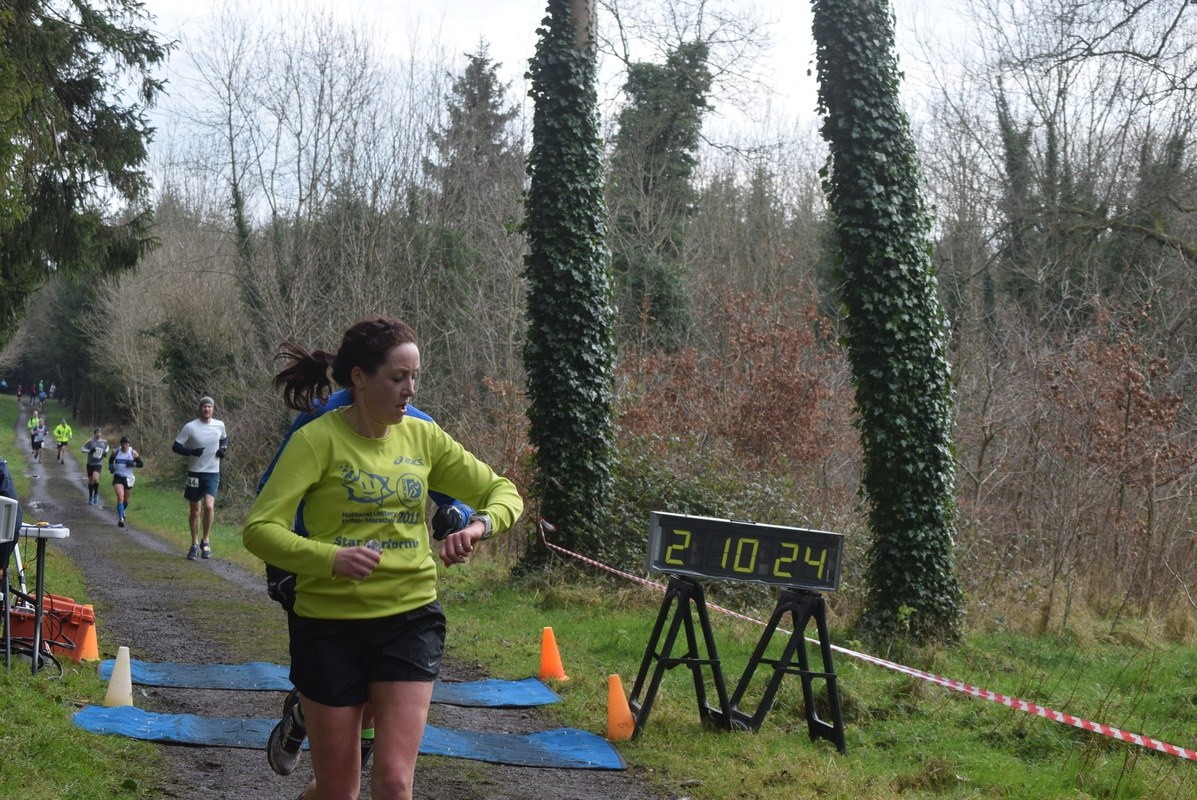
470;512;494;541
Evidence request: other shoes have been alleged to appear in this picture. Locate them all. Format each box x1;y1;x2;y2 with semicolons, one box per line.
61;461;64;464
32;450;34;453
39;459;42;463
57;456;61;459
35;454;38;458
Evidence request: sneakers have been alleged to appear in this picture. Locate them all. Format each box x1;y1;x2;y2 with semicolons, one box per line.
89;497;93;505
200;538;211;559
118;518;125;526
94;494;98;503
266;687;307;776
360;728;375;771
187;545;200;560
124;509;126;517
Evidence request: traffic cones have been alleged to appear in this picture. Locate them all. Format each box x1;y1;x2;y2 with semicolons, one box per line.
101;646;135;709
540;626;572;684
603;673;640;744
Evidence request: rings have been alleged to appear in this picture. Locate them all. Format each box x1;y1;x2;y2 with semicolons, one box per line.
455;544;462;547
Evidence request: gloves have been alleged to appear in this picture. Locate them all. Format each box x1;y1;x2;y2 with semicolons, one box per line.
126;460;134;467
109;467;115;473
216;448;226;458
34;431;38;435
44;432;47;435
432;505;464;541
191;447;205;457
90;448;96;453
103;452;106;457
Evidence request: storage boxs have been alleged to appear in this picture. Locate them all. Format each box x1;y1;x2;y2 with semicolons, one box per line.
10;595;96;662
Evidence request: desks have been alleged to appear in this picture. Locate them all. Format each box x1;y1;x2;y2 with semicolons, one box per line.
18;526;70;675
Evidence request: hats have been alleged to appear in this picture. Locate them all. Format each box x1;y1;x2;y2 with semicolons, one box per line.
61;418;65;422
94;428;101;433
120;437;130;443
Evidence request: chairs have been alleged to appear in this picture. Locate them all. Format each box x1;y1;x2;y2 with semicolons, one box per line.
0;496;18;667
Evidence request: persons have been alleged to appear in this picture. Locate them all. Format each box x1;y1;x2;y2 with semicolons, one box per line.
53;417;73;464
255;389;477;775
243;318;524;800
29;379;57;413
0;457;23;624
80;428;109;505
16;384;25;402
109;436;143;527
27;410;42;454
30;418;50;463
172;397;228;560
0;379;8;394
